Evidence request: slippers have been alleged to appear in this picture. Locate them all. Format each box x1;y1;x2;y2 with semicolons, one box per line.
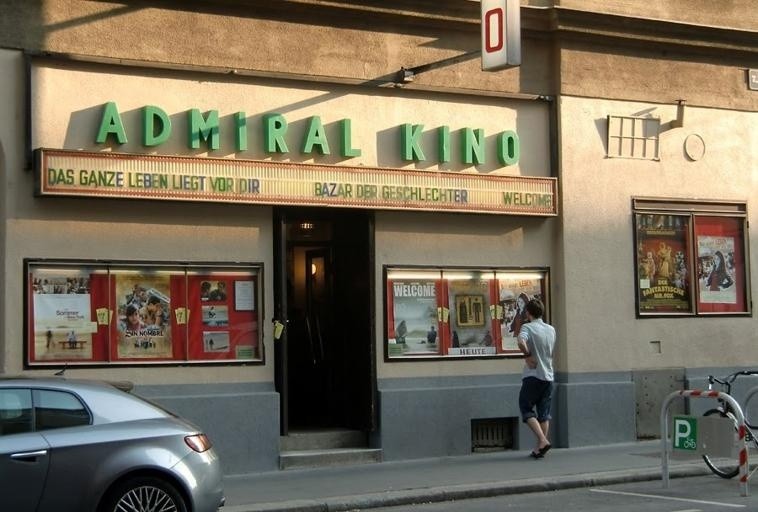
536;444;551;458
530;452;544;458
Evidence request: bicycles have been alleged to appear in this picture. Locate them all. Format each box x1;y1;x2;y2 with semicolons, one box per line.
695;370;757;480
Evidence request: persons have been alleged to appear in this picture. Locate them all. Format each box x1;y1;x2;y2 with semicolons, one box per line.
451;331;460;347
33;276;228;351
478;330;492;347
509;292;530;338
396;321;409;338
517;299;557;459
427;326;437;344
706;250;734;292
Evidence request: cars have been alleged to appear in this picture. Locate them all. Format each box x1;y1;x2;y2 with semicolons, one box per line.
1;363;228;512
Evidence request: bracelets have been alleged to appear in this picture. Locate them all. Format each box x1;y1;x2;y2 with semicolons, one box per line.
524;351;531;358
